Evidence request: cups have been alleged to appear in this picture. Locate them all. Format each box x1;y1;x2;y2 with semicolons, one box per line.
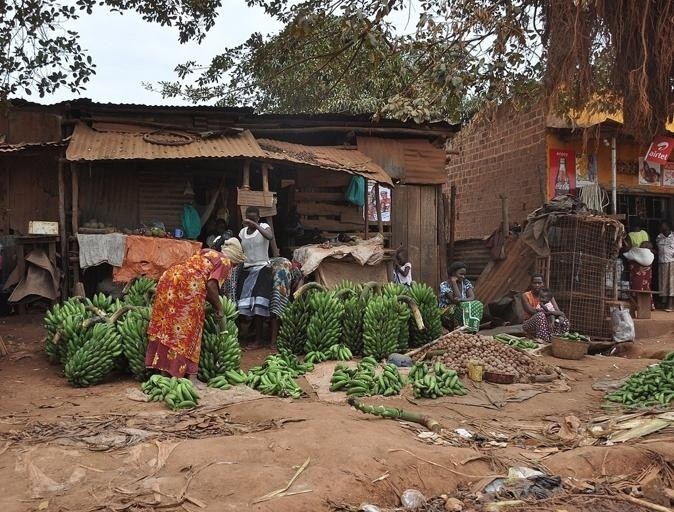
175;228;184;238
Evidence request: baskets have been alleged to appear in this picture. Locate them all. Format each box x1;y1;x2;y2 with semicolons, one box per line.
550;333;589;359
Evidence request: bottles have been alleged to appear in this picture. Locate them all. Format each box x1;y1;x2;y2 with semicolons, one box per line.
552;156;571;197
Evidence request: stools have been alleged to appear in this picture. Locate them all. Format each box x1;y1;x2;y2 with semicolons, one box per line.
619;288;664;319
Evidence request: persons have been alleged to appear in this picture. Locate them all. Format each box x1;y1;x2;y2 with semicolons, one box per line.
436;261;483;333
535;287;557;332
144;236;245;378
520;274;570;342
391;248;411;286
205;206;303;351
655;218;673;311
622;217;649;249
627;240;656;311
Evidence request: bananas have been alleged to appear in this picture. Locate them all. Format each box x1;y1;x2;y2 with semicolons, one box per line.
603;352;674;407
42;275;467;411
560;332;590;341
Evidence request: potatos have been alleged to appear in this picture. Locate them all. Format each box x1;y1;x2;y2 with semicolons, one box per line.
81;218;168;239
411;330;561;380
